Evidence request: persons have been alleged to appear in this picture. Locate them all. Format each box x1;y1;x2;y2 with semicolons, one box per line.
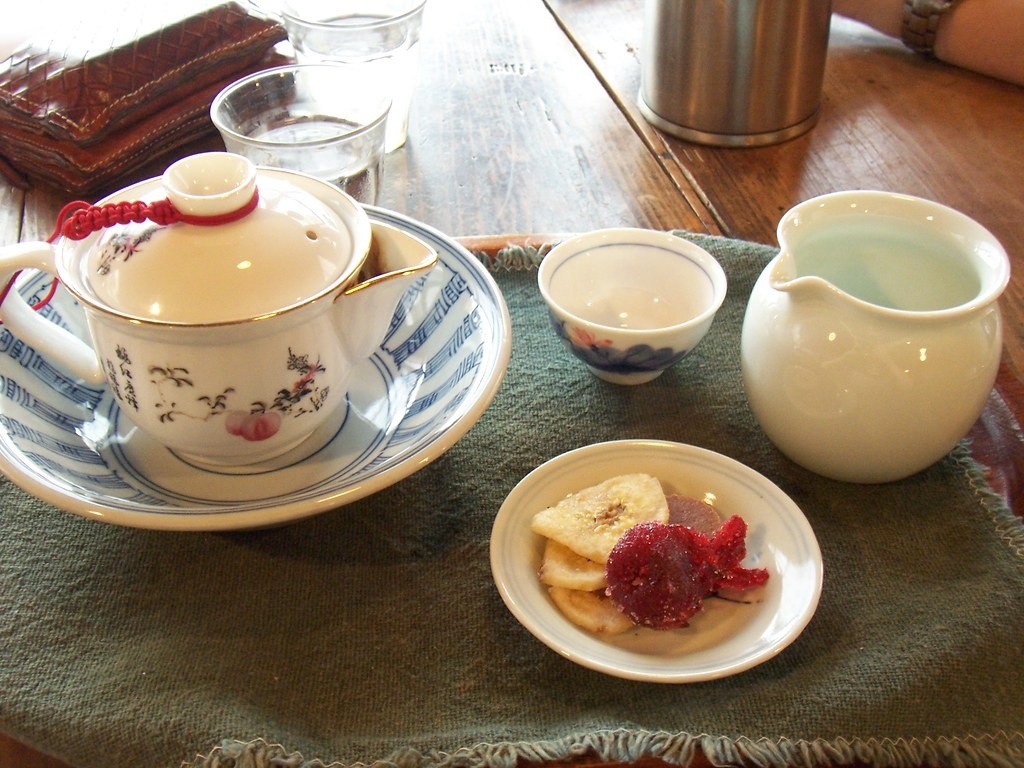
831;0;1024;86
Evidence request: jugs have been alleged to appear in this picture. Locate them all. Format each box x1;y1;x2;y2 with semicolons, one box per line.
739;189;1013;484
0;151;435;465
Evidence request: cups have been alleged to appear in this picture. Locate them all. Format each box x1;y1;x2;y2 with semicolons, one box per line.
280;0;428;154
209;63;396;205
538;228;727;384
637;0;833;147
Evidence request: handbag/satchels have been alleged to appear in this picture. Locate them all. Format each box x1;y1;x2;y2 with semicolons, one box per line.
0;1;298;195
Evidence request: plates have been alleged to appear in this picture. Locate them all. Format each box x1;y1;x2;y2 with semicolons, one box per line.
489;439;826;684
0;201;512;530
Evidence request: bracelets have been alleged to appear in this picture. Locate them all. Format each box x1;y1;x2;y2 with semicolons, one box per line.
902;0;960;65
